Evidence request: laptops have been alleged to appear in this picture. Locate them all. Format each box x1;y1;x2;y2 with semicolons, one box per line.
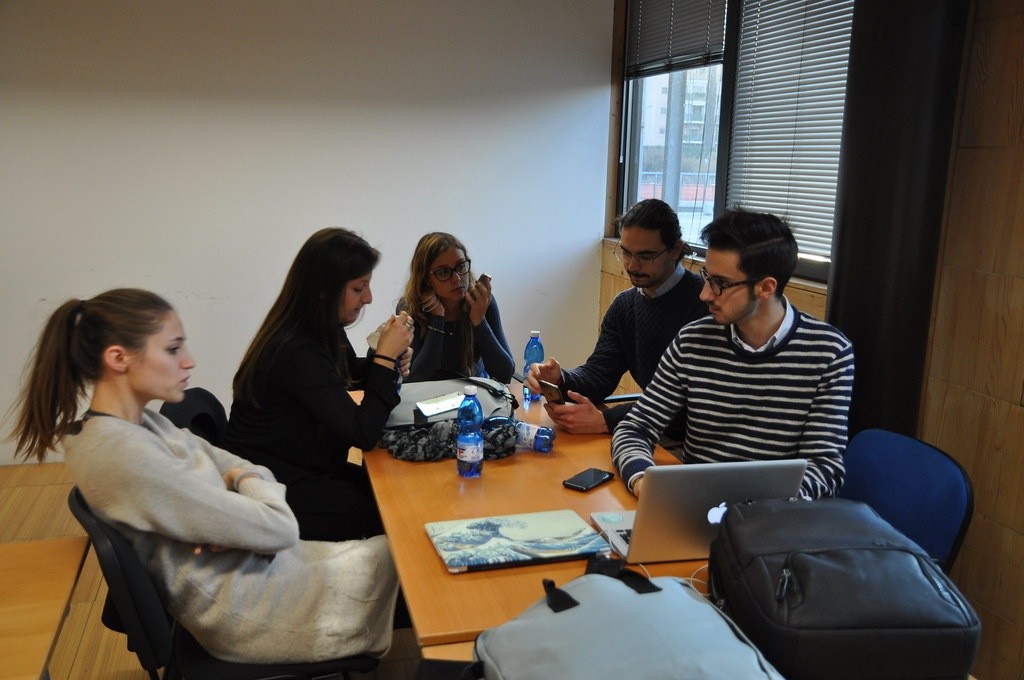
425;508;611;574
590;460;808;565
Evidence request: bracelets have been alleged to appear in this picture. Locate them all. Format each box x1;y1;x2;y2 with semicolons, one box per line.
234;471;263;490
420;294;439;312
632;475;643;495
428;326;445;333
374;354;397;363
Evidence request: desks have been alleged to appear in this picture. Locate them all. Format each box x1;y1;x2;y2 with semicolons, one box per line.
0;536;91;680
346;382;710;660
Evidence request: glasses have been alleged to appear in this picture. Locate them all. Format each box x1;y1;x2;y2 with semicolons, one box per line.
612;237;675;267
429;257;471;281
699;266;766;295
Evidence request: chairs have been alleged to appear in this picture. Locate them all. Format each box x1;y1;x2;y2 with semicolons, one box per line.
68;482;379;680
841;429;974;572
160;387;227;450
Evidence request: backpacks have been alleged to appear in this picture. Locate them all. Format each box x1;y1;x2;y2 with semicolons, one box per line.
707;497;982;680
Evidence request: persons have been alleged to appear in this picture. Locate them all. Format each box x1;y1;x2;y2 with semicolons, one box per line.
9;288;411;665
225;227;415;544
526;198;713;460
396;232;515;386
610;204;855;502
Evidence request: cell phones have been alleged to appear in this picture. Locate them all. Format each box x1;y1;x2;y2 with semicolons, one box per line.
416;390;465;416
539;380;565;406
563;468;614;492
463;274;492;307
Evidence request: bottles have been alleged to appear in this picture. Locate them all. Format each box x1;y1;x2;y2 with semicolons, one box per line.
480;416;556;453
522;331;544;401
456;385;484;479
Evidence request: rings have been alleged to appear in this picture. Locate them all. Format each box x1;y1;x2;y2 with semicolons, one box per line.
405;321;412;327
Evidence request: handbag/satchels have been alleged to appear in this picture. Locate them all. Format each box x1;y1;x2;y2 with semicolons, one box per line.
472;555;786;680
